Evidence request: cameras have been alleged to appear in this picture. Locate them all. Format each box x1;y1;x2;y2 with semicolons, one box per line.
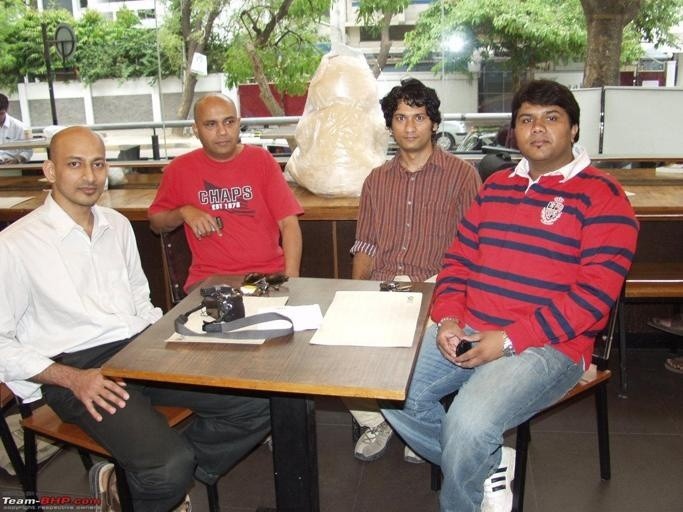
200;284;245;322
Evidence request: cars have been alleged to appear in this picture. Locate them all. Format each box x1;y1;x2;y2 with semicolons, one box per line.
457;128;520;156
29;125;108;143
239;121;468;160
106;135;201;159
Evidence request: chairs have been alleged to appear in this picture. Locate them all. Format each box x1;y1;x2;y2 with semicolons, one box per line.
2;135;294;159
0;384;30;484
15;360;217;511
429;269;626;512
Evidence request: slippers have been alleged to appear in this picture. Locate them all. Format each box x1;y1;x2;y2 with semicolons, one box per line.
648;315;682;336
665;356;682;374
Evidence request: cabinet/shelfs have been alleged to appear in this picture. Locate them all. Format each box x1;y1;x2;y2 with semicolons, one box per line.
2;182;682;315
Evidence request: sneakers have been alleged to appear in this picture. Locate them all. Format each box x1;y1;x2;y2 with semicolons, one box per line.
174;493;192;512
480;446;516;512
403;445;428;464
88;460;121;511
353;420;393;462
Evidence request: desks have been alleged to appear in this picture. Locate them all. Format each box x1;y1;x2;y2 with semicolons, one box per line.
104;269;437;511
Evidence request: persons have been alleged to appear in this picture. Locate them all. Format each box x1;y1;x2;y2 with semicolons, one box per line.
0;124;272;511
375;78;641;512
339;76;484;466
145;93;307;453
0;92;33;166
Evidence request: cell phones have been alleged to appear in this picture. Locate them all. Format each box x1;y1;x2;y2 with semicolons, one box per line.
198;215;224;235
456;340;471;357
380;281;399;290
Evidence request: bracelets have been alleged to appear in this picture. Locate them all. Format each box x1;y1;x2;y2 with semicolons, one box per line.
499;329;514;357
433;316;459;341
